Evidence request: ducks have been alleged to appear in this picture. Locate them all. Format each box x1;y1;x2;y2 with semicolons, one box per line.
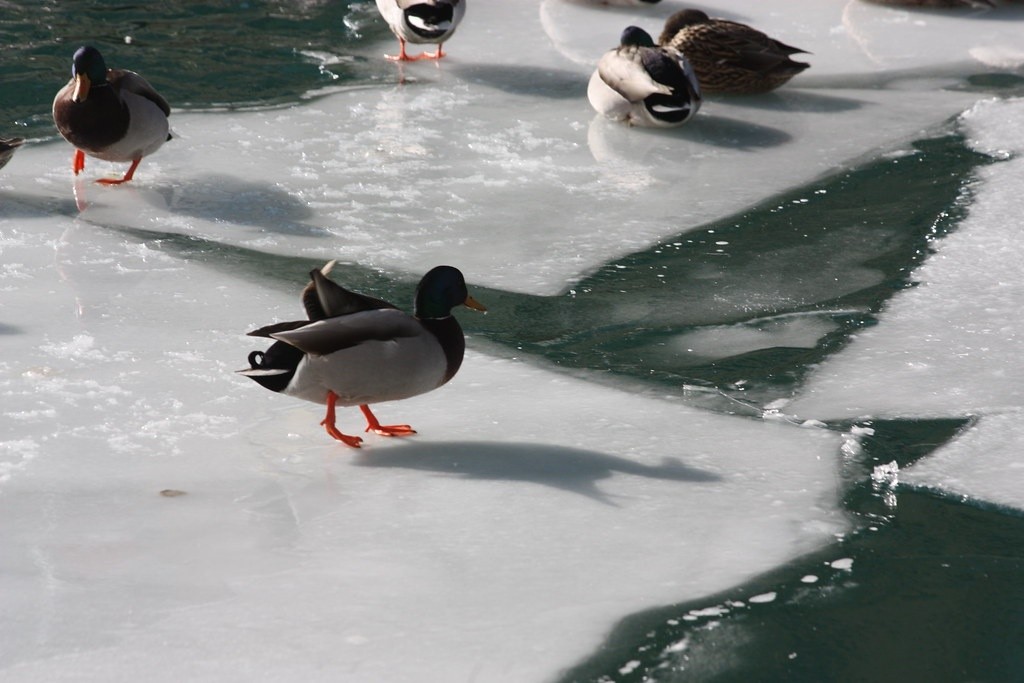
656;9;811;95
587;26;703;127
51;46;181;184
235;258;487;449
376;0;466;61
